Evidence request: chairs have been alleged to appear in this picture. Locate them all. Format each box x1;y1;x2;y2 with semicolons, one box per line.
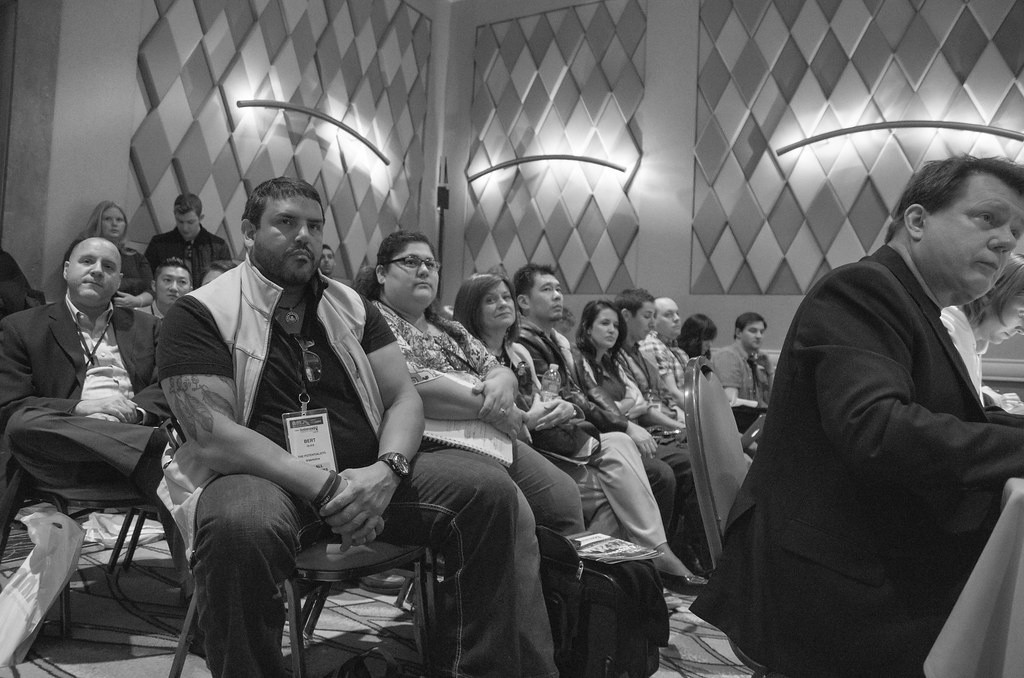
14;404;439;677
684;353;771;678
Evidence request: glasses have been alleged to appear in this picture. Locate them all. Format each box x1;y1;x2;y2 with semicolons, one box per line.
293;333;322;383
382;255;442;272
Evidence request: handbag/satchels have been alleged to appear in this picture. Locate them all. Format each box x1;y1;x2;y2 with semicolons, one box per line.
537;526;670;678
0;511;86;667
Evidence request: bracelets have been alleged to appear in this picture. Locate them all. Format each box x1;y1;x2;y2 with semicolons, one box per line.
137;295;143;307
313;470;342;507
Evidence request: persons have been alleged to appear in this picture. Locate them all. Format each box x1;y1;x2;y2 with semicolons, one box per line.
0;237;208;659
353;265;382;303
689;153;1024;678
369;229;586;678
157;178;560;678
133;257;193;321
570;288;776;575
938;252;1024;417
63;199;154;307
143;192;232;291
452;264;710;596
318;244;353;287
198;260;245;285
513;263;695;546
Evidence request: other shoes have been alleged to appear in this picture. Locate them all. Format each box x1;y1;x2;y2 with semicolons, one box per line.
358;574;405;595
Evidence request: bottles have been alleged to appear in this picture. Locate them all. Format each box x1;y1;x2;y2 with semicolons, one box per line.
540;364;562;403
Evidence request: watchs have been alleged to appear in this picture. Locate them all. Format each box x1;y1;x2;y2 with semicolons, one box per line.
378;452;411;479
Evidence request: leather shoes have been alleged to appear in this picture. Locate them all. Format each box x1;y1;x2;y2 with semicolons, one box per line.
657;569;709;595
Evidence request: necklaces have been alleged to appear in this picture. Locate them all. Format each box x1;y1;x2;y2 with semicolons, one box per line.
278;294;305;325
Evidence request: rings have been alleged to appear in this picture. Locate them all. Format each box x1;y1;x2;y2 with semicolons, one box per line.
500;408;506;413
553;424;555;427
